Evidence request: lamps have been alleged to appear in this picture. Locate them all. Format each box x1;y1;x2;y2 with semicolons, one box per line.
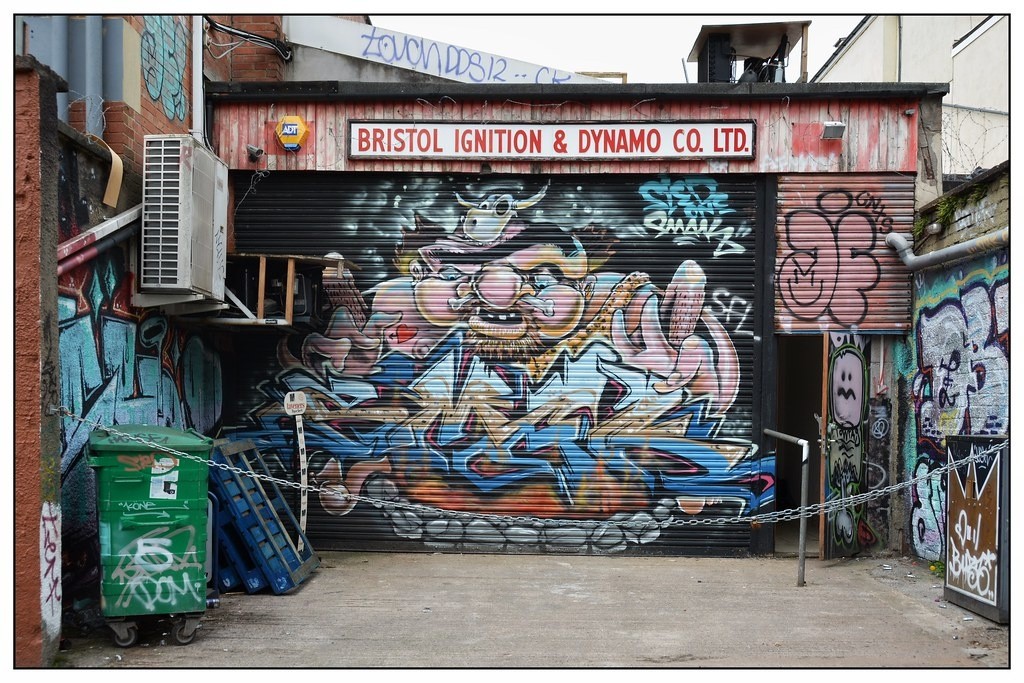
819;121;847;141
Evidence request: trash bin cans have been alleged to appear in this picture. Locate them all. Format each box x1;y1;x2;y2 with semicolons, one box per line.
84;425;215;648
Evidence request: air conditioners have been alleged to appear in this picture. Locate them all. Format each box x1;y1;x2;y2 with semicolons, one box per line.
138;132;229;304
